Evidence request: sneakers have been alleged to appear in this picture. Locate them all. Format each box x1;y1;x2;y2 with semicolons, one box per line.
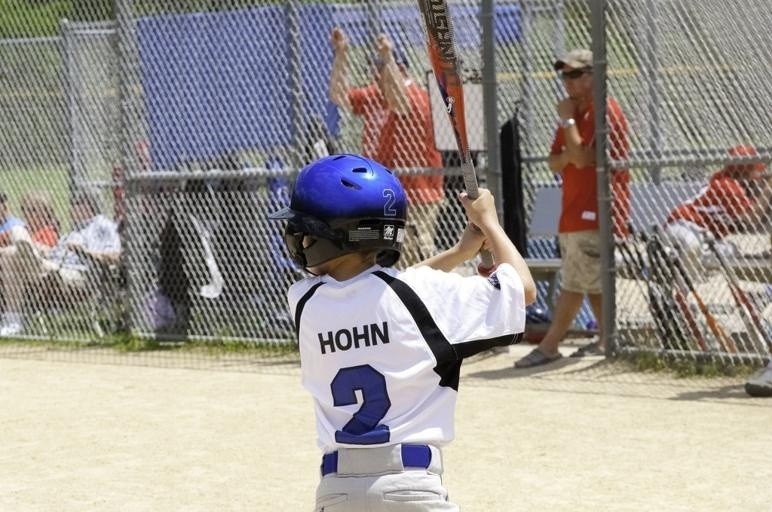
1;311;24;336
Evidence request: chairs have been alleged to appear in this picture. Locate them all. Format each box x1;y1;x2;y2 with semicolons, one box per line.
33;216;124;337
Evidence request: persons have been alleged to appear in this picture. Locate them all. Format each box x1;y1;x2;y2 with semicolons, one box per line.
516;49;629;367
648;145;772;365
0;187;218;339
270;114;332;335
263;151;538;511
327;27;447;274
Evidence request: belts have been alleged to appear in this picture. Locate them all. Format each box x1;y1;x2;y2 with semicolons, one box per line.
320;444;431;477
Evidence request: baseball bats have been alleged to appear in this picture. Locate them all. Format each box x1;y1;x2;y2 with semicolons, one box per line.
614;223;772;367
416;0;496;276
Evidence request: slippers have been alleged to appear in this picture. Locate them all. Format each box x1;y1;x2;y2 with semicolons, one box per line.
515;347;562;368
570;341;605;356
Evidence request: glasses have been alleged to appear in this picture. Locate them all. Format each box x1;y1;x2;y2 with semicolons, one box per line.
559;71;592;78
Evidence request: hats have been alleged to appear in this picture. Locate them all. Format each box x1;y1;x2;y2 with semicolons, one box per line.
555;49;593;71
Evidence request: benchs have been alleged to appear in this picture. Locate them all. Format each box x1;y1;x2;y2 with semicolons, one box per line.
521;255;772;342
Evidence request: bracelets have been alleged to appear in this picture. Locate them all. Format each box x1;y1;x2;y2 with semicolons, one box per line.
559;118;576;129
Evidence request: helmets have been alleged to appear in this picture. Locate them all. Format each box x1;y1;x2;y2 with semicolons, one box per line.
268;153;408;267
